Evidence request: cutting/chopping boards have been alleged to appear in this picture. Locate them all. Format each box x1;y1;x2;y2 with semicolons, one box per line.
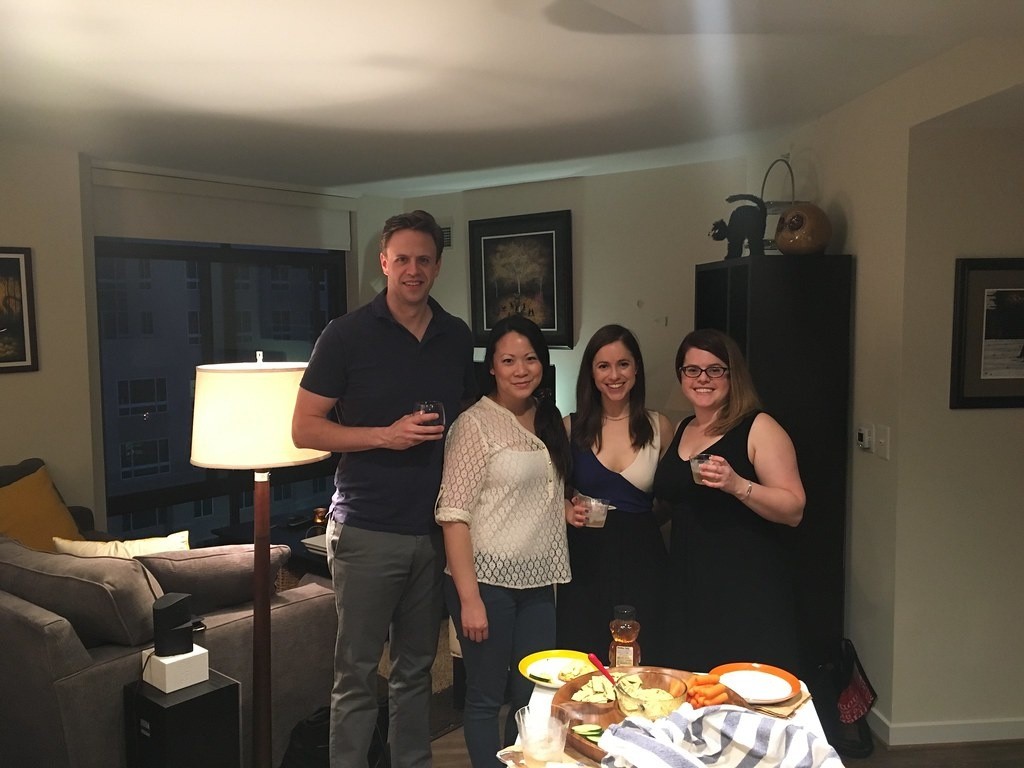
550;653;704;762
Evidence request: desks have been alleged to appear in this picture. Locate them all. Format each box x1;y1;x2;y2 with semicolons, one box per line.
212;503;333;580
506;672;828;768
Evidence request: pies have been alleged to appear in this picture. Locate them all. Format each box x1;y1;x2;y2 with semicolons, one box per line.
558;665;591;682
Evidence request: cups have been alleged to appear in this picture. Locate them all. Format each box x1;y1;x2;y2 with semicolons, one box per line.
690;454;717;484
413;402;446;435
515;703;570;768
582;498;610;528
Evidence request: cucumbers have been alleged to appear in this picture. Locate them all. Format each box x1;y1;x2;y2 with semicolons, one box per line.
572;724;603;744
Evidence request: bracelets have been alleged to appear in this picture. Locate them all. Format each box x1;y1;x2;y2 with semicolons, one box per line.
738;481;752;501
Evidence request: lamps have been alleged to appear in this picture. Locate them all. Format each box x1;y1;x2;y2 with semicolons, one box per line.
189;351;331;767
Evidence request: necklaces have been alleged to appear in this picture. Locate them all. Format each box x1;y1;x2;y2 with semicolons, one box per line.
605;415;629;421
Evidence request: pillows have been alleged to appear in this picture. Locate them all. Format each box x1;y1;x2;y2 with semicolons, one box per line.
133;543;292;616
0;457;87;552
53;528;191;559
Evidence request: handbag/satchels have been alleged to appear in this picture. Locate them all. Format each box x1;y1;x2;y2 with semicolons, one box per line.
279;699;389;768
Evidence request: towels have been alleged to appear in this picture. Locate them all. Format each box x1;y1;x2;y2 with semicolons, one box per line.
596;699;845;768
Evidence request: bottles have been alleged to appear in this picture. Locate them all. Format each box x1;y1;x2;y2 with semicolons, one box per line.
609;606;641;667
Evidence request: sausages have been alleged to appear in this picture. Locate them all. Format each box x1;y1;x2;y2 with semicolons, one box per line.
685;674;729;709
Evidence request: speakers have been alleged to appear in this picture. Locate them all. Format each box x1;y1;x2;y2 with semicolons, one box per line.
153;592;194;656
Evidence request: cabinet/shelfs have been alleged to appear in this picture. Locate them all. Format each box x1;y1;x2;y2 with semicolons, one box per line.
695;254;853;680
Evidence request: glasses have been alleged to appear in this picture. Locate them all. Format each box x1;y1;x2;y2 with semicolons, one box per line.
679;365;729;378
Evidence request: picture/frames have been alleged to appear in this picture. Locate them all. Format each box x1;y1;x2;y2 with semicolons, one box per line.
468;210;574;350
0;247;39;372
950;258;1024;410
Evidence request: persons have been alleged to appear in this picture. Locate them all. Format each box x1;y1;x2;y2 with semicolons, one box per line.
561;325;674;669
653;328;806;677
435;316;573;768
292;209;477;768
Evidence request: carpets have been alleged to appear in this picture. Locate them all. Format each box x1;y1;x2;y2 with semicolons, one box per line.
301;573;512;741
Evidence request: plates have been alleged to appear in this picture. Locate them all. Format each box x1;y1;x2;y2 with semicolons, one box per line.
518;649;599;689
710;662;801;704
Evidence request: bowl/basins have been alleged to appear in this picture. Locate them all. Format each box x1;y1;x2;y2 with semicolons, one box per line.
615;672;688;721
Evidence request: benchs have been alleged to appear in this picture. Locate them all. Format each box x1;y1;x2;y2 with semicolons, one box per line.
1;506;339;768
